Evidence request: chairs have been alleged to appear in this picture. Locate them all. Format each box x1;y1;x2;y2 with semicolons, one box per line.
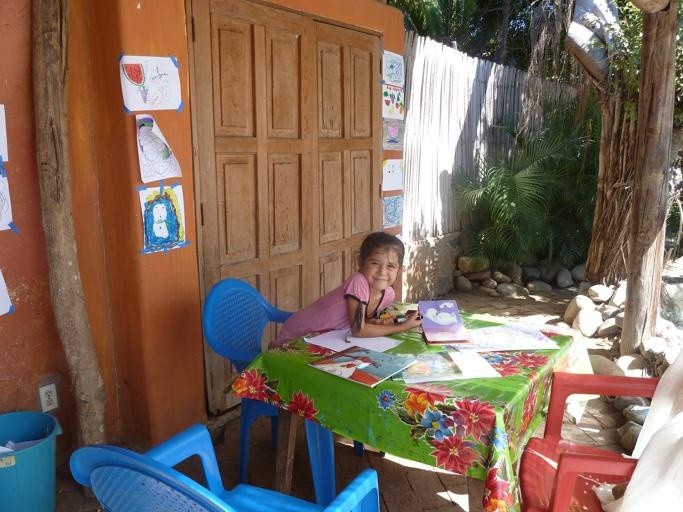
69;418;385;511
196;279;366;470
522;359;680;512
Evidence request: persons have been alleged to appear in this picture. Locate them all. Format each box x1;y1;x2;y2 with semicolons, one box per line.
269;232;423;348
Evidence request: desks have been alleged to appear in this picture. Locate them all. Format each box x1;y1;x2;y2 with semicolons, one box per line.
233;295;574;510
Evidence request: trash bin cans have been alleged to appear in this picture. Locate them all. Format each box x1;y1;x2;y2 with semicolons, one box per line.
0;411;64;512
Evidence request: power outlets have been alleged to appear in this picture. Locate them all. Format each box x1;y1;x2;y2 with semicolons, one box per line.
38;383;59;414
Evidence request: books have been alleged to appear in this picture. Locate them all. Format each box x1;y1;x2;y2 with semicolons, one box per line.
307;346;418;389
419;300;472;345
